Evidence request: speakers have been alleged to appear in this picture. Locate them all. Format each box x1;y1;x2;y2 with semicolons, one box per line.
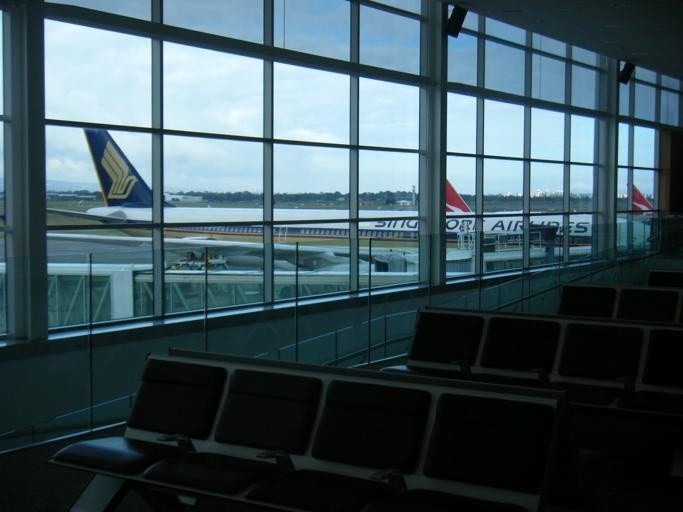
619;62;634;85
444;5;470;39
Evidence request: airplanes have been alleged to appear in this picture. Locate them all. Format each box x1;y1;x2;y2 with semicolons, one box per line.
413;179;655;221
46;128;652;270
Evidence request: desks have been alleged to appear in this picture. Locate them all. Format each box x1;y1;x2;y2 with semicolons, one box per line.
456;230;542;251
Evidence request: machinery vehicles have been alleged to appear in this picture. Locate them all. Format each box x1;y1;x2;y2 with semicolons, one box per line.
169;250;230;272
